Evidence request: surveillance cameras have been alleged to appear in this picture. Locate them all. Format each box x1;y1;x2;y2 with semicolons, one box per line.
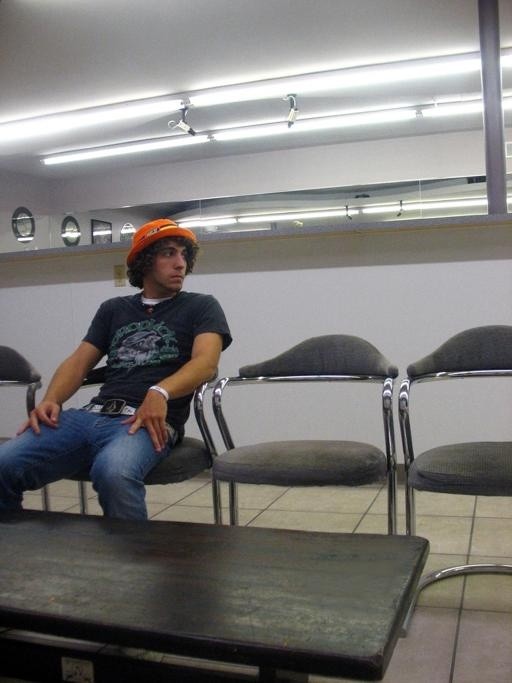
287;108;298;124
178;121;196;137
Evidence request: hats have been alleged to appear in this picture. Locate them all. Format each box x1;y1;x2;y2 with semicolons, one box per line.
123;219;197;272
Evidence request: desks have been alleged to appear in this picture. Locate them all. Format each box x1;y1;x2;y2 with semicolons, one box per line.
0;509;432;682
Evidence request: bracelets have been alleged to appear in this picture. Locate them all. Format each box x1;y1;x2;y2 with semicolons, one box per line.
147;386;171;401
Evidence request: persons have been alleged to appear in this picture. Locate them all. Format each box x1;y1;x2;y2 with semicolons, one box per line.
1;217;232;522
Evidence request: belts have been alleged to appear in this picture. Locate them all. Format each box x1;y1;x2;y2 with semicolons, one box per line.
84;399;179;446
